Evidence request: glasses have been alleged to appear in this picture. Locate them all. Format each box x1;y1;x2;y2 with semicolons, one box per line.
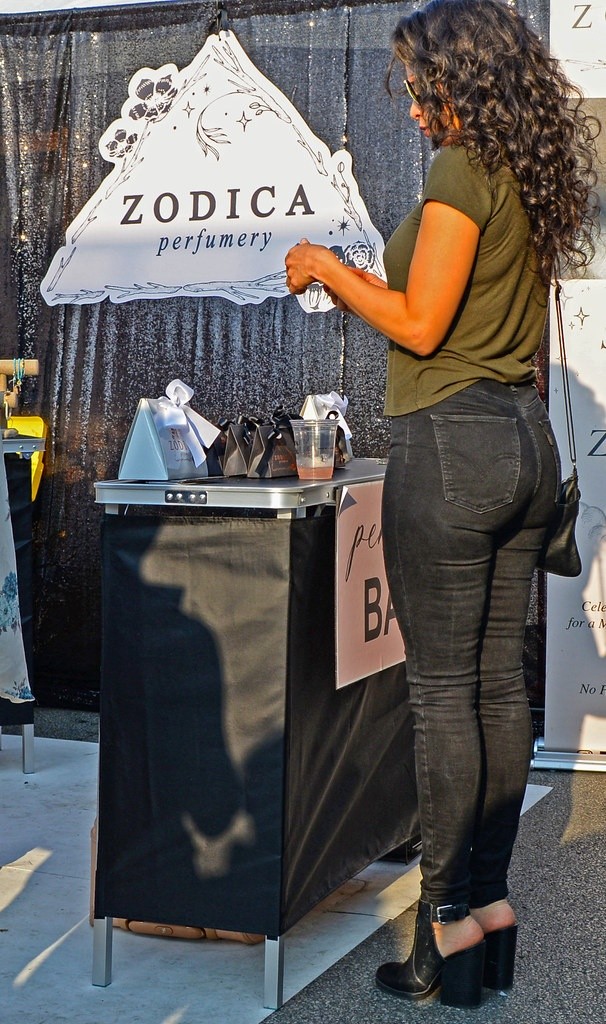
404;73;422;107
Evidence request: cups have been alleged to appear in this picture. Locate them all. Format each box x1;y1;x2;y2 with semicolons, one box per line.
289;418;339;479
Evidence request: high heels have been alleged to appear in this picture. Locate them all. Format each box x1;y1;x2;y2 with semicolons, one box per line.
374;899;518;1008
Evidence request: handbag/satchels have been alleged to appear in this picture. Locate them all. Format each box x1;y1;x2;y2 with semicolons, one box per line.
533;467;581;577
223;416;264;479
246;413;304;478
202;418;230;477
116;380;220;481
298;391;355;467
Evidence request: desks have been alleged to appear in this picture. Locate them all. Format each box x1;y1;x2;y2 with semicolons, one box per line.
89;456;391;1008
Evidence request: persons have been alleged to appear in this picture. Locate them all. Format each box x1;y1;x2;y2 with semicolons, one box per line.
284;1;603;1009
